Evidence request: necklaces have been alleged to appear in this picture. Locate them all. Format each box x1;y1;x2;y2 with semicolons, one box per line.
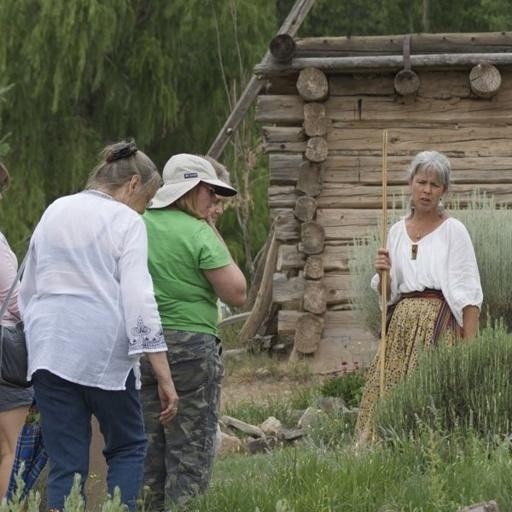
407;218;441;242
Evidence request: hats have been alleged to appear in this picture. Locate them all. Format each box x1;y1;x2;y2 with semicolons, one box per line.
147;154;238;210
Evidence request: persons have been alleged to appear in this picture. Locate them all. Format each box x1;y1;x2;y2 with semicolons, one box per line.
198;155;233;231
140;153;247;512
18;137;180;512
354;148;484;450
0;158;36;506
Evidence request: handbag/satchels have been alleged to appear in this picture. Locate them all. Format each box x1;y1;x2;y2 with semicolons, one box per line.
0;322;31;388
